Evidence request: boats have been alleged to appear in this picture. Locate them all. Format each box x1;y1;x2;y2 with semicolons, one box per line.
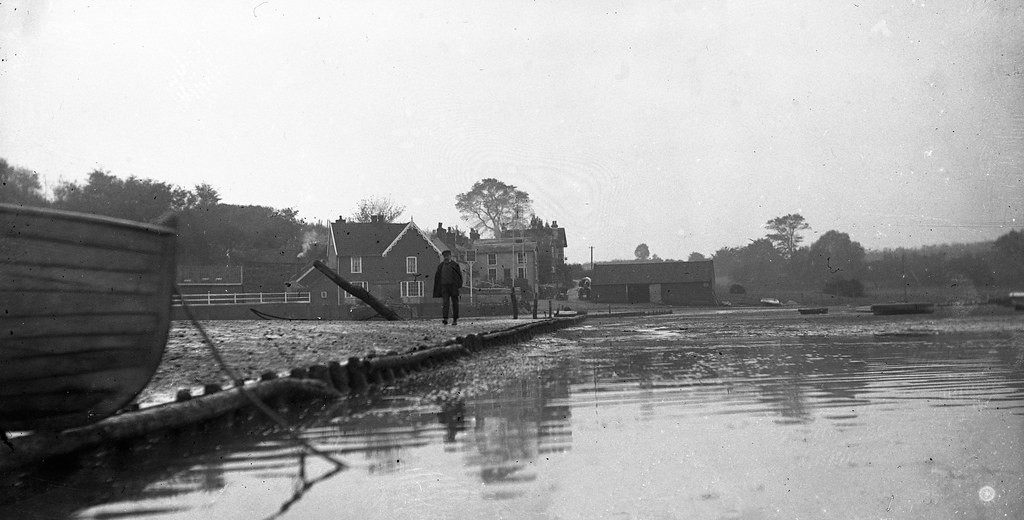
1008;292;1024;310
760;297;780;305
0;203;181;441
554;310;578;317
871;301;935;316
797;307;829;314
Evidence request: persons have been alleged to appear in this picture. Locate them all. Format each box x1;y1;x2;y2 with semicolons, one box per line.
433;250;463;325
579;281;583;286
503;296;508;302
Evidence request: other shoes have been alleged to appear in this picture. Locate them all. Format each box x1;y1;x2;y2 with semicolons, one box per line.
452;322;457;325
442;320;447;324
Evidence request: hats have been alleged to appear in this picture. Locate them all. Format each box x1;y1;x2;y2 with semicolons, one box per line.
442;250;451;255
